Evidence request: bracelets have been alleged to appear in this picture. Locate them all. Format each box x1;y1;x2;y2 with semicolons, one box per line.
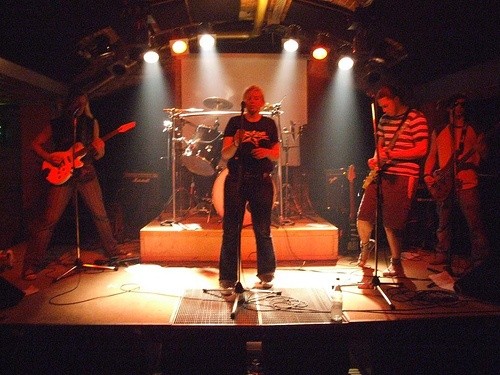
232;140;238;147
423;174;433;177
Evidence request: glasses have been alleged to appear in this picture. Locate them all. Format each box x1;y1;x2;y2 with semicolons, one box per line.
454;101;465;106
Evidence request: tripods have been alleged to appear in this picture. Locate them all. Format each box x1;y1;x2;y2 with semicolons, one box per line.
335;132;404;311
203;134;283;319
51;120;119;283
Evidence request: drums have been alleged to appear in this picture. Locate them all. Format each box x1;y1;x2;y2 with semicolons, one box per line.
210;165;278;228
182;124;226;176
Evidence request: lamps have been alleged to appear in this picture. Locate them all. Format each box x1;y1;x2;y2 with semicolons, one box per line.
279;23;384;84
107;23;217;80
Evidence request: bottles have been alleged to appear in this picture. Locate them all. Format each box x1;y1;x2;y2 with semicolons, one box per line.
330;278;343;321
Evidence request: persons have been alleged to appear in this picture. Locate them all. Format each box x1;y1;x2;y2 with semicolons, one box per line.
355;86;429;276
424;94;491;264
21;87;133;281
220;85;281;295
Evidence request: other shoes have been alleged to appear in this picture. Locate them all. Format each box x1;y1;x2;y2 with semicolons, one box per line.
429;256;445;265
357;239;375;267
25;273;36;280
261;279;275;288
219;281;233;295
110;250;132;258
383;262;404;276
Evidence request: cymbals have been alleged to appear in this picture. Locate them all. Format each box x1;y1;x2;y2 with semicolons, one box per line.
200;97;233;111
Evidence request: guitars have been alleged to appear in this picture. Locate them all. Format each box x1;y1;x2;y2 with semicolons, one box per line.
40;120;137;186
337;164;362;257
428;132;486;202
361;147;389;190
